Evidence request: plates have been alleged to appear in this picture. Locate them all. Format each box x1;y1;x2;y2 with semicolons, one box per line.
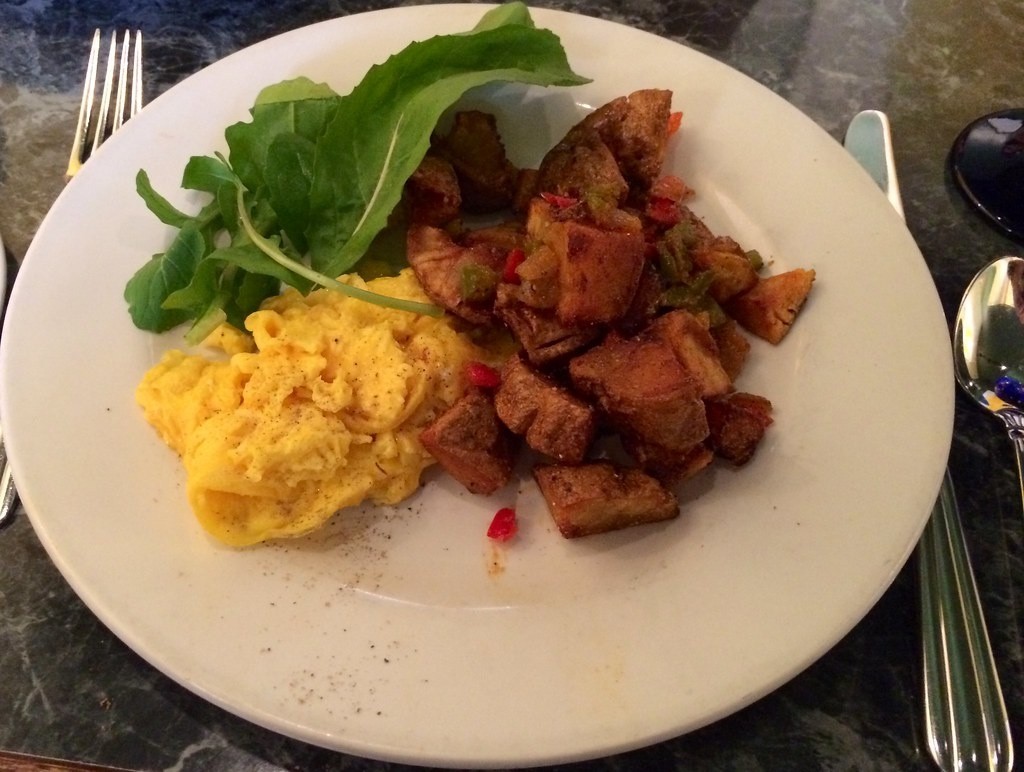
0;3;954;769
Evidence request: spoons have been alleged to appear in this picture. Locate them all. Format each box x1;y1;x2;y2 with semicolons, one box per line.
955;255;1024;524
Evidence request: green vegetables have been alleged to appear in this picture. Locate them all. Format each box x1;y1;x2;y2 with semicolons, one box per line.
121;1;594;347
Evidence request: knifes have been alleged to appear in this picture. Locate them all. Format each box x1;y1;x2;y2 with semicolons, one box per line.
840;111;1016;772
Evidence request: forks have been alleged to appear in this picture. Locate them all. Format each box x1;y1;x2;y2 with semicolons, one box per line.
0;27;144;528
